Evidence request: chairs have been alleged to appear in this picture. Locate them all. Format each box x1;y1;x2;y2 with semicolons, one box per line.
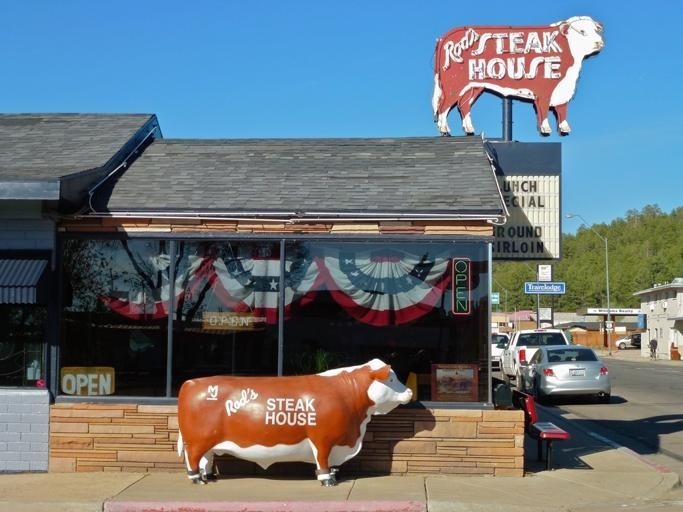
549;355;561;362
518;337;561;345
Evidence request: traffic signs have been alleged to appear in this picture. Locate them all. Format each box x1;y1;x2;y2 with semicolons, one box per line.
523;282;565;294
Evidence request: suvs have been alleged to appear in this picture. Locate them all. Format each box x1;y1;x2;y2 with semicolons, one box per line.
499;330;571;378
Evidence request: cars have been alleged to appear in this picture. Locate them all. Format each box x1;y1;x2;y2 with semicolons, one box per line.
517;345;611;401
492;333;510;369
616;334;641;349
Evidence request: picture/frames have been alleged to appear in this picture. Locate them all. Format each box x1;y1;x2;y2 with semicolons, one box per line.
429;363;479;401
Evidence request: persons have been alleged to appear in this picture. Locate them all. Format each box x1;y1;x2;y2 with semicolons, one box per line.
647;337;657;359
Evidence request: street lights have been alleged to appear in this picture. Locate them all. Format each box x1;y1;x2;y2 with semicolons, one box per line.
566;214;613;355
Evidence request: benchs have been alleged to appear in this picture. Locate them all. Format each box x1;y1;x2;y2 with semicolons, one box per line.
511;389;570;472
